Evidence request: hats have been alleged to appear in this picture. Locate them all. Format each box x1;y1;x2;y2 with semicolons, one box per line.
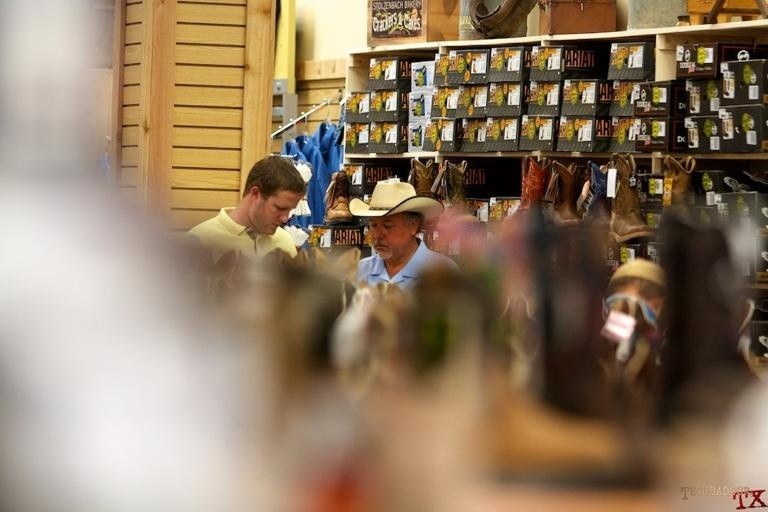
605;257;668;293
348;178;444;224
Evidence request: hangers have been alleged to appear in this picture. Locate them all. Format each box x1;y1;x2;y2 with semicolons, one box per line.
274;97;332;157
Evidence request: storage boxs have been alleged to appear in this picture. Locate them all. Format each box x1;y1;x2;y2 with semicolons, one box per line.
537;0;617;35
367;0;460;49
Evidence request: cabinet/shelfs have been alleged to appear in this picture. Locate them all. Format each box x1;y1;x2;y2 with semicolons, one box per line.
342;19;767;290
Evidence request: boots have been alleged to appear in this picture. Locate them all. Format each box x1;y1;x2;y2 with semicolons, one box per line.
606;151;658;244
324;170;355;226
504;153;551;225
543;160;585;230
410;157;445;208
443;160;481;226
661;153;697;225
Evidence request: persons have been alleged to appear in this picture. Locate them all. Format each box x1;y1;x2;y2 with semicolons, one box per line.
347;179;460;294
184;156;307;261
165;204;768;511
